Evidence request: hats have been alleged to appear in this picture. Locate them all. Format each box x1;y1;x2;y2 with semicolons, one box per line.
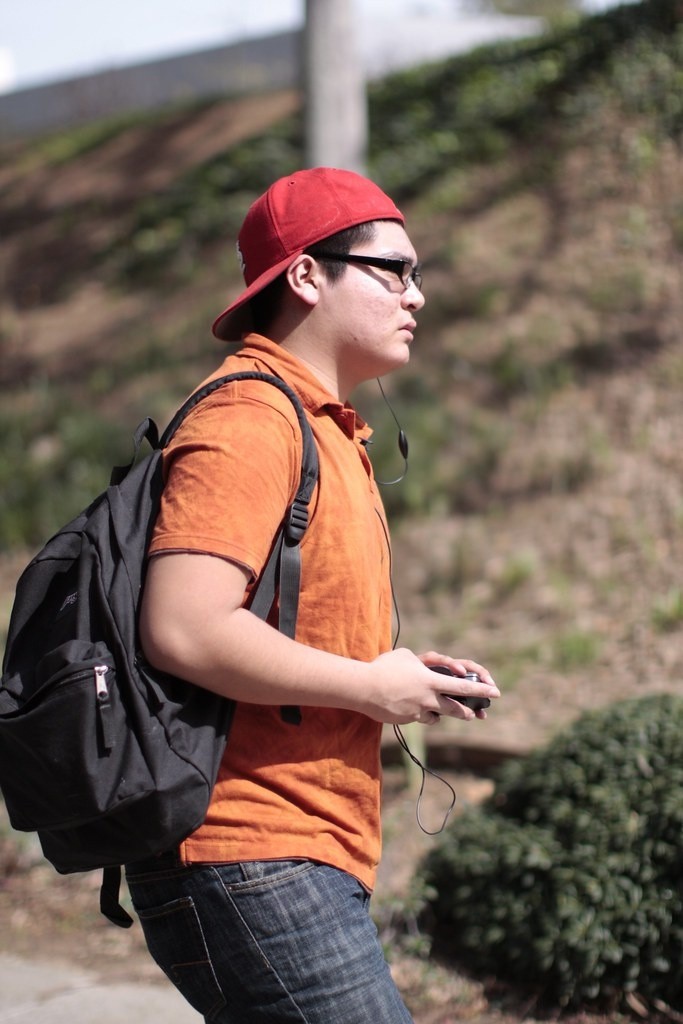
211;167;405;342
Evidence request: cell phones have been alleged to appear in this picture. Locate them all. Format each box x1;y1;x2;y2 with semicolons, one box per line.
443;673;491;712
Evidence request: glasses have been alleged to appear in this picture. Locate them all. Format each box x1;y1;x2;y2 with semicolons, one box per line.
304;251;423;290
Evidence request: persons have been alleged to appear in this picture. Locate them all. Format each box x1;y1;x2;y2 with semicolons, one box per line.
120;168;500;1024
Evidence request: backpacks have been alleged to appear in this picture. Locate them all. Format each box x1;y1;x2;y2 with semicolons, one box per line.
0;371;318;875
